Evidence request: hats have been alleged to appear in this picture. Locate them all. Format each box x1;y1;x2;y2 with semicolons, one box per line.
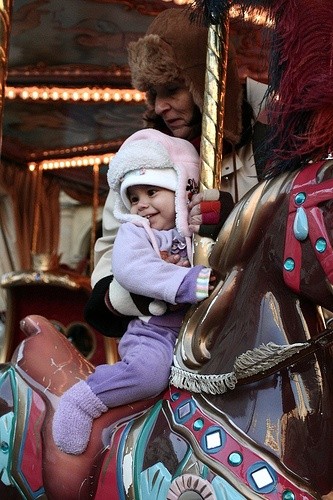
120;167;178;211
107;128;199;239
128;8;242;145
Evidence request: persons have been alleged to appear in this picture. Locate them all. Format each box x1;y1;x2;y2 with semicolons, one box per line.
51;129;217;455
86;9;278;338
